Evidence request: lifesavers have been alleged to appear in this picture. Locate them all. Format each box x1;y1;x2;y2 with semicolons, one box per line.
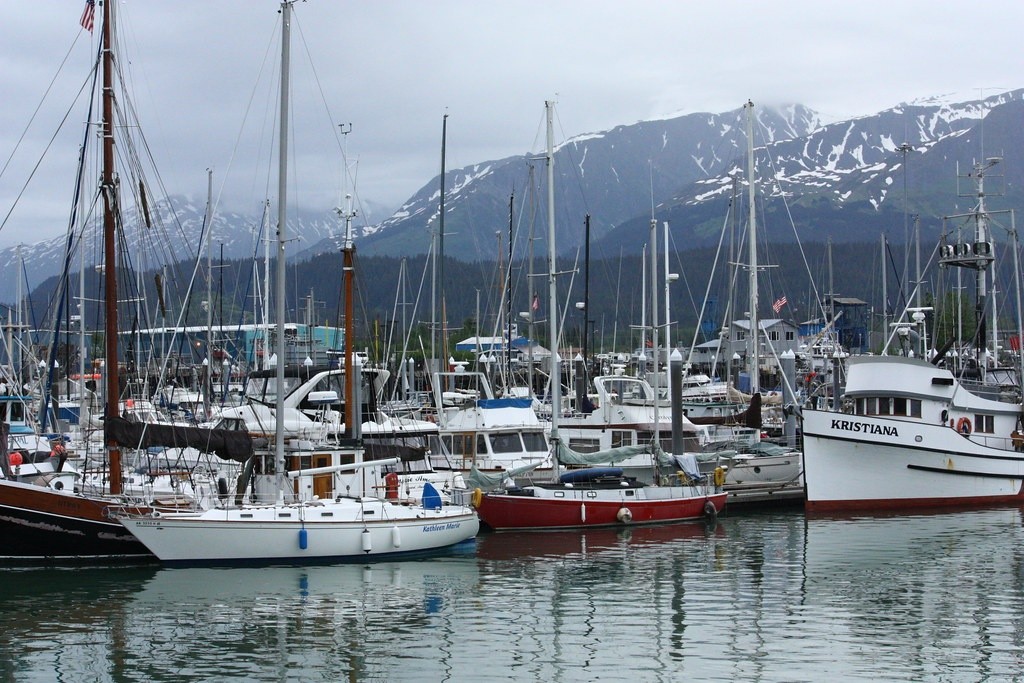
957;417;971;437
473;488;481;508
714;467;724;487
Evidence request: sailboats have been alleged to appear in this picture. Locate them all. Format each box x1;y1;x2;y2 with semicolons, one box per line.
1;0;1024;566
